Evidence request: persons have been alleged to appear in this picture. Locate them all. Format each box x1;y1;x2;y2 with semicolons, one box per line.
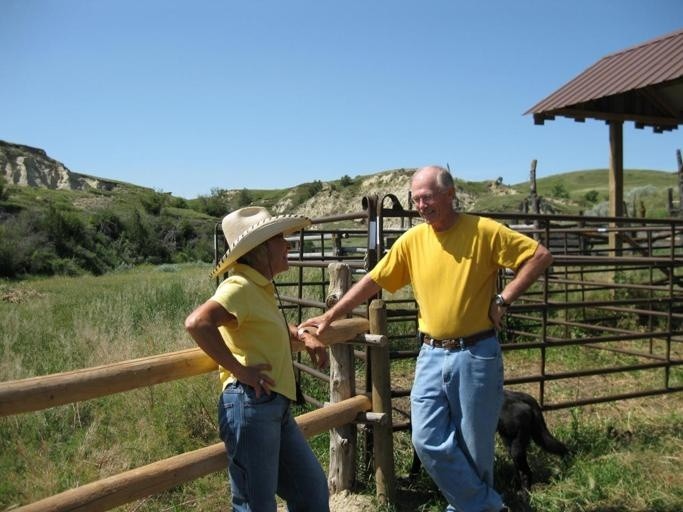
302;166;554;512
181;205;331;510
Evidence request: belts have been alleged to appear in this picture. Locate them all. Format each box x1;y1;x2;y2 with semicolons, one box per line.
424;330;495;352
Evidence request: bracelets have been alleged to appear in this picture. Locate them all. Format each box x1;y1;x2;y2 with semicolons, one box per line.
298;327;309;334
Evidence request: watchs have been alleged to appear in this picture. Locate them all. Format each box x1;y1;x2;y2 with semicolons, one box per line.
493;294;512;308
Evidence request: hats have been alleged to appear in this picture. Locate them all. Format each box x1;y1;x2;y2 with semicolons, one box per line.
209;206;313;277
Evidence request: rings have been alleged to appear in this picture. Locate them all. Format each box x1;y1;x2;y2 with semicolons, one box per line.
258;378;264;384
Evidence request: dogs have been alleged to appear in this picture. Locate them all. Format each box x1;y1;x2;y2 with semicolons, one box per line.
411;390;569;506
